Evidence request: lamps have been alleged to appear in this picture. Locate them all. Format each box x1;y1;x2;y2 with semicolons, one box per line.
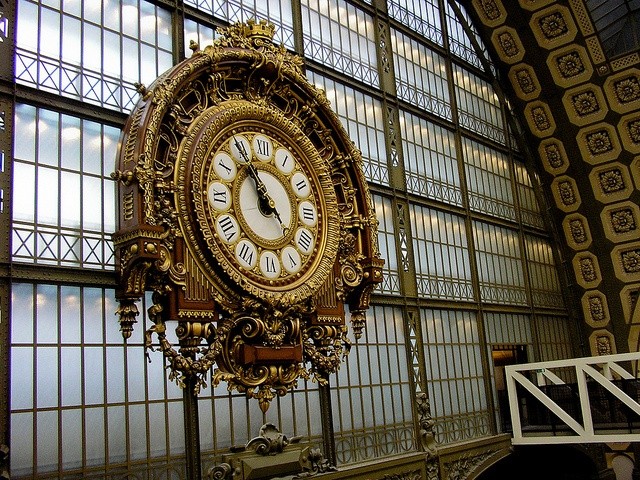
109;17;385;413
